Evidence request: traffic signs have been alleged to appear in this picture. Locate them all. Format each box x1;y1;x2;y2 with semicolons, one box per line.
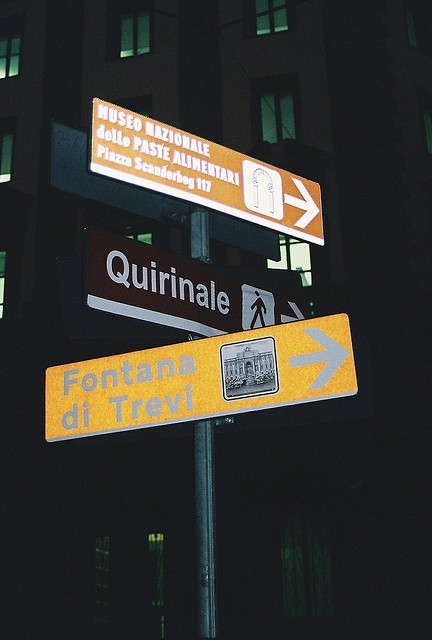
86;224;313;338
45;313;359;441
90;95;324;248
